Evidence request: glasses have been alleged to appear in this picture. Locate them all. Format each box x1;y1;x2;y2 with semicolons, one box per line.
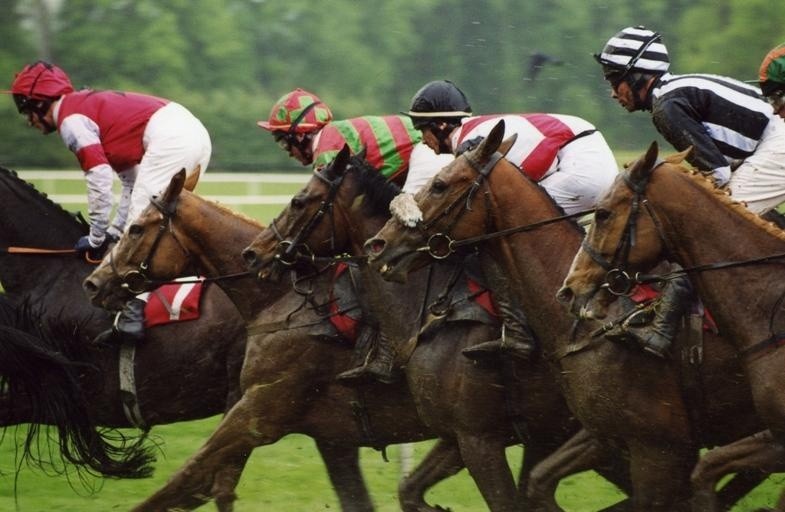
764;90;785;115
608;78;628;93
277;133;294;152
19;105;34;122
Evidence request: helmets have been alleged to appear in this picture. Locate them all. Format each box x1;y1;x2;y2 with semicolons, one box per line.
592;25;669;75
745;43;785;86
0;59;74;101
399;79;472;119
257;88;333;134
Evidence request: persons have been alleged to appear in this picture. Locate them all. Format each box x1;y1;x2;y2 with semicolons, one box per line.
589;24;785;365
258;89;458;390
745;43;784;124
401;79;621;365
12;61;214;347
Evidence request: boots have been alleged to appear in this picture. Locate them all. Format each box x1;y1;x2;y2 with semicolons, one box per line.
336;321;403;387
461;249;536;364
624;260;697;359
92;292;152;348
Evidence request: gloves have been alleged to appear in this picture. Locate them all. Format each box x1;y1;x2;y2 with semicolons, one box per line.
74;236;93;252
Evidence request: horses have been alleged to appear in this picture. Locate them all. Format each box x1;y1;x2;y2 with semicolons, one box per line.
552;139;785;511
238;140;630;512
1;167;375;512
359;117;765;509
78;164;437;512
